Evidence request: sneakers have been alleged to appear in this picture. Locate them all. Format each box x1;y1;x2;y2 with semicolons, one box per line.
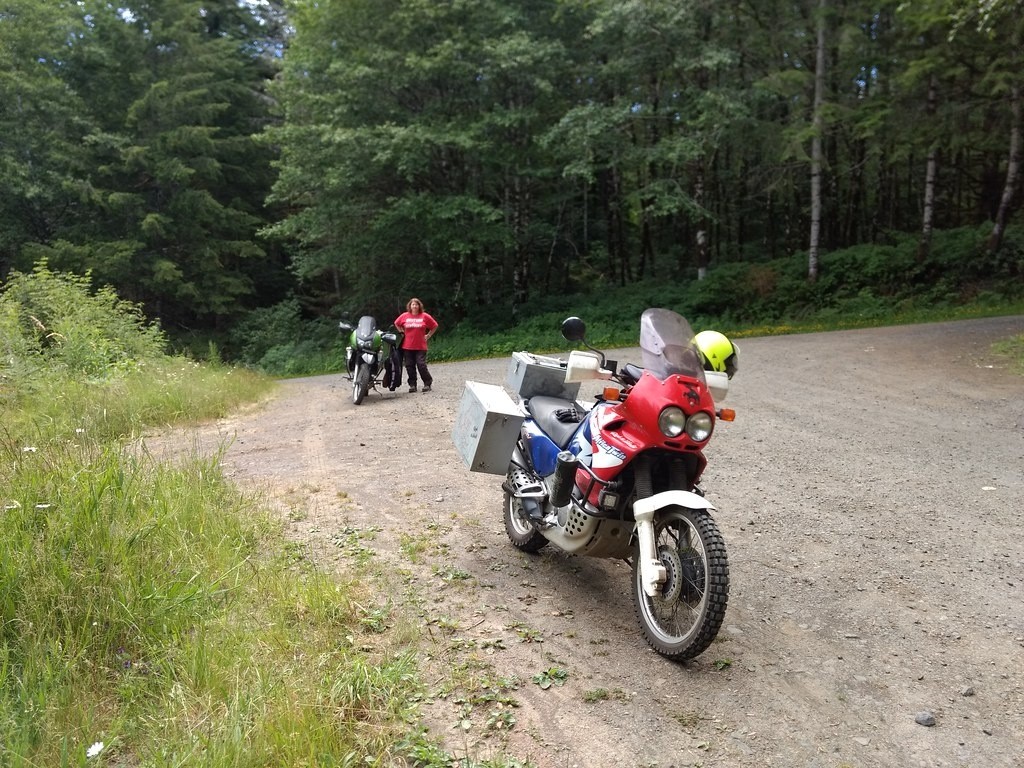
422;385;432;392
409;386;418;393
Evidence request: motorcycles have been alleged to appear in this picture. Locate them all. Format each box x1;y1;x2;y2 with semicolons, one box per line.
451;306;732;660
340;315;398;404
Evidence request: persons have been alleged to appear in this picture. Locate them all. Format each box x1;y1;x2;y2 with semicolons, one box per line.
394;298;438;393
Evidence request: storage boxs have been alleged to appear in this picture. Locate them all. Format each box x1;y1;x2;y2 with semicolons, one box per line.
507;352;582;402
450;381;526;476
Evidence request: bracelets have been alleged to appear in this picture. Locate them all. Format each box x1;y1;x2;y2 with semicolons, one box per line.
427;334;431;338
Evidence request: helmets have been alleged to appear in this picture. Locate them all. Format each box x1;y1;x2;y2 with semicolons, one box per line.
688;330;738;380
